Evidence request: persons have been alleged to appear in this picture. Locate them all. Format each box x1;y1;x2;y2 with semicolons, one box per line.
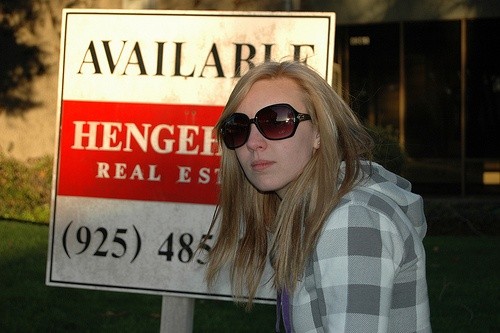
191;54;431;333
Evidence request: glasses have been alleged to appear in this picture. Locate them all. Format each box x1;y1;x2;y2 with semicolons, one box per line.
217;102;312;150
